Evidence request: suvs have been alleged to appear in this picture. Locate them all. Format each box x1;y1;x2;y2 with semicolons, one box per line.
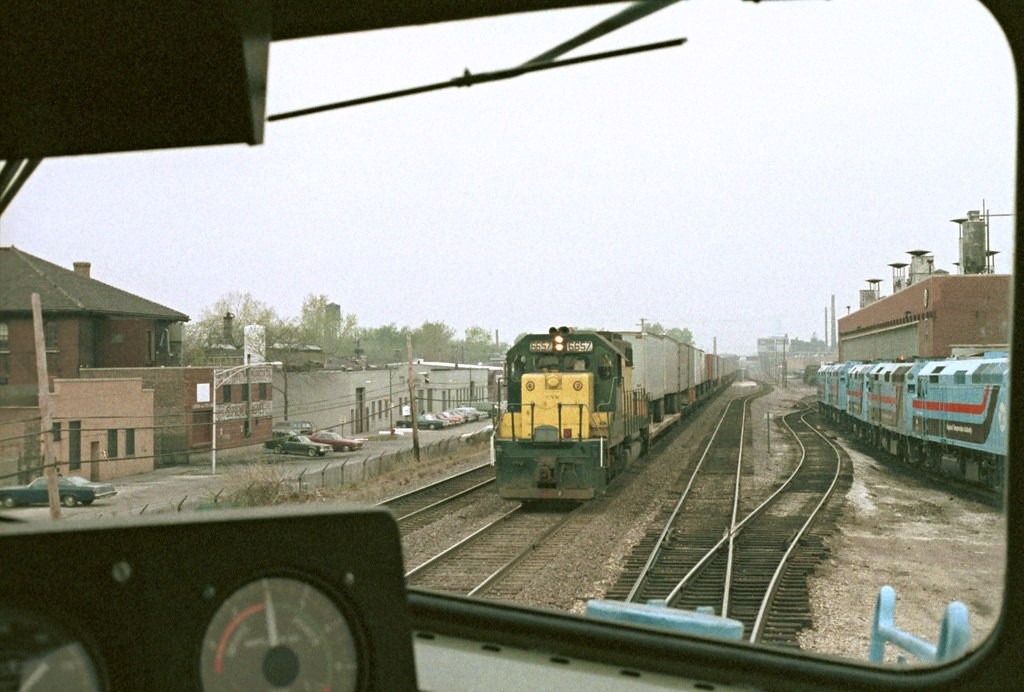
272;421;314;437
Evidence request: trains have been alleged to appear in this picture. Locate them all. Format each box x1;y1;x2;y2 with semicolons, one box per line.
491;328;736;500
816;351;1009;495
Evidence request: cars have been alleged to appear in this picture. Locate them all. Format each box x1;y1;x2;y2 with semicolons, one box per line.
264;435;333;456
0;476;117;508
433;407;488;427
308;431;364;452
396;415;448;430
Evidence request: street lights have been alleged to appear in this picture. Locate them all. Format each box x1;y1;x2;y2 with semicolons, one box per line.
211;361;283;474
